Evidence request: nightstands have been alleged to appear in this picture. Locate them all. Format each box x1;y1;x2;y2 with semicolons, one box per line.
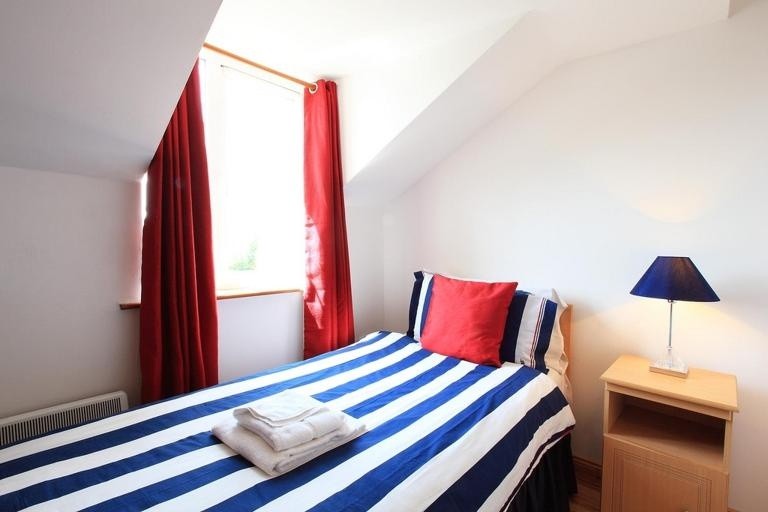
601;353;740;512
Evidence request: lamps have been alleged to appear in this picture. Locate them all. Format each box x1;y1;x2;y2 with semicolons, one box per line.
629;256;720;379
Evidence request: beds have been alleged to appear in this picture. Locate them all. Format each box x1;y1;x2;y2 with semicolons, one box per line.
0;332;578;512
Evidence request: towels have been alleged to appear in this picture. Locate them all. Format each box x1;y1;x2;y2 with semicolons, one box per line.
212;390;366;476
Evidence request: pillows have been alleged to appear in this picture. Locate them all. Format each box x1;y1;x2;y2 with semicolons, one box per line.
419;274;518;368
407;271;557;369
518;285;569;375
